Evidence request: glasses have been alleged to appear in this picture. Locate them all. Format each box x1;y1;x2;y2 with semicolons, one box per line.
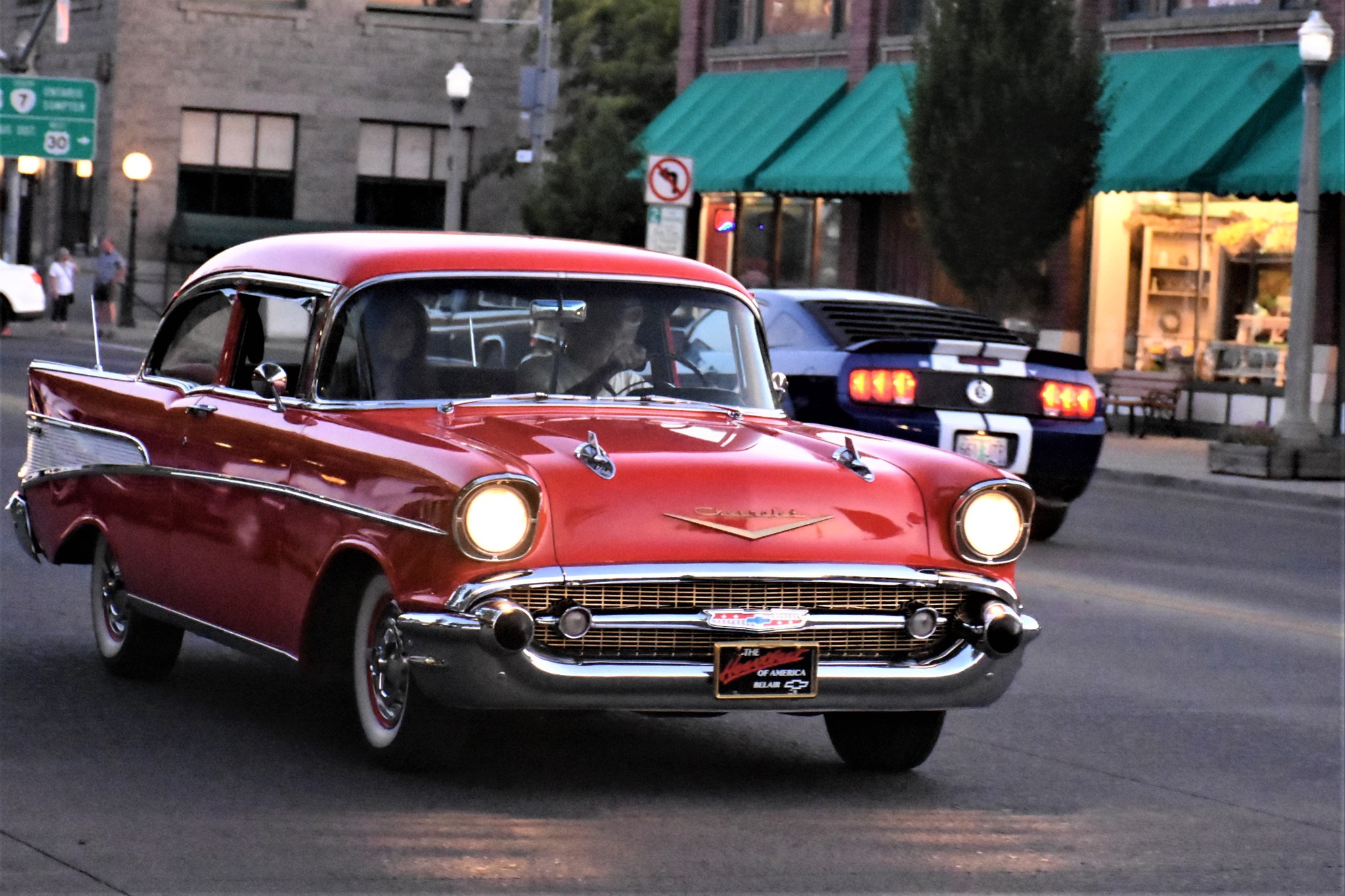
620;305;643;327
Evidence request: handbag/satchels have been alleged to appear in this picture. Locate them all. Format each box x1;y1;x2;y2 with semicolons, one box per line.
69;292;74;304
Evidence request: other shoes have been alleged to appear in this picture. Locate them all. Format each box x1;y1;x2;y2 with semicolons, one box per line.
98;328;116;340
51;320;66;331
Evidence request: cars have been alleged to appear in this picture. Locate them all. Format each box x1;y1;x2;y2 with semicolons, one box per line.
421;286;538;368
632;287;1107;541
0;259;46;334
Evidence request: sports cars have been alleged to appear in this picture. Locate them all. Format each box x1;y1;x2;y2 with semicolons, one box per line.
5;229;1044;774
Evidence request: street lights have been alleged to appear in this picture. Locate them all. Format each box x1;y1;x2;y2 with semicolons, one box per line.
443;55;474;233
1271;1;1335;447
116;152;152;327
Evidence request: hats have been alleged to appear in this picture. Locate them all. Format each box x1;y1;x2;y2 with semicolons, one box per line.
58;247;69;259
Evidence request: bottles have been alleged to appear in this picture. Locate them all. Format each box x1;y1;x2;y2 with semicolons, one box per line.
1151;277;1158;291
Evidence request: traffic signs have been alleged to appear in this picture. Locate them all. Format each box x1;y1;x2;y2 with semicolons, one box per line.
0;114;98;162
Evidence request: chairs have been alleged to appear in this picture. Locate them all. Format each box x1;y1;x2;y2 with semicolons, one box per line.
325;291;435;402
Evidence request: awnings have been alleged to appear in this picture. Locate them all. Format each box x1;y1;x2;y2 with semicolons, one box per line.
754;41;1304;194
626;69;848;191
1211;49;1345;199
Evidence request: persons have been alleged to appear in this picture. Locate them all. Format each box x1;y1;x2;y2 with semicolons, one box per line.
92;236;125;339
327;293;447;403
46;247;77;335
517;288;655;397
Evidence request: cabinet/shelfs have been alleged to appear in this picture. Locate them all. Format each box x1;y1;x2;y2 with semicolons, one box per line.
1136;224;1232;363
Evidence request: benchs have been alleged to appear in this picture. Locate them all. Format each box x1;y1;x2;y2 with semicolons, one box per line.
1103;367;1185;437
181;360;514;400
